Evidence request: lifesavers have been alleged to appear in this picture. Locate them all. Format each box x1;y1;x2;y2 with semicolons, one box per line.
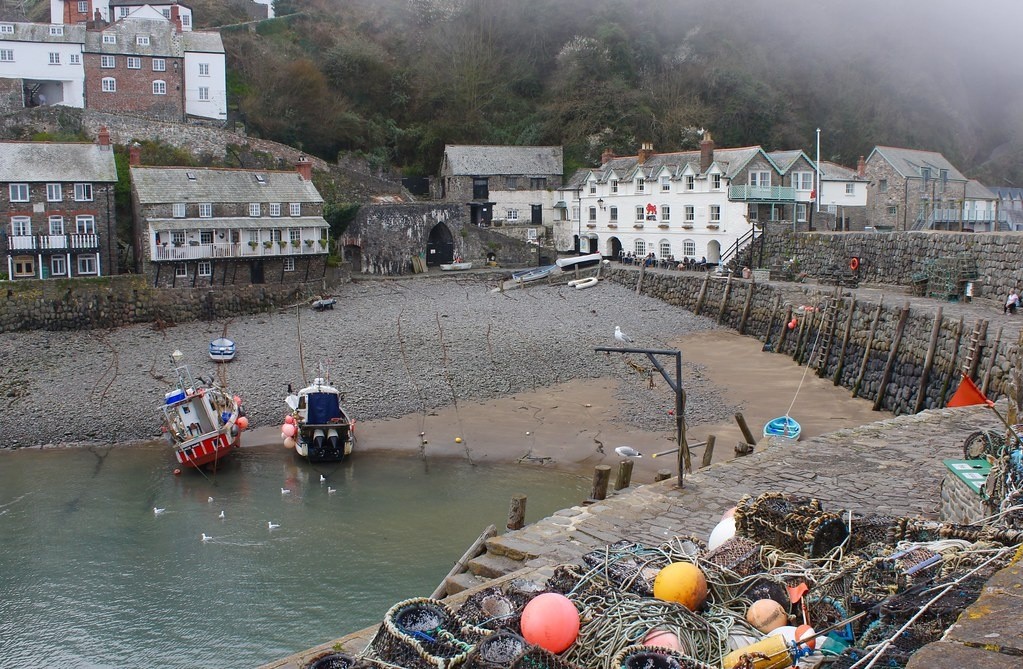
850;258;859;270
351;418;355;433
293;419;297;436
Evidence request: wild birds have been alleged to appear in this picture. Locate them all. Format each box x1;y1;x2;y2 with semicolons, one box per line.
208;497;214;503
320;475;326;481
268;522;280;528
201;533;214;541
281;488;290;494
219;511;226;518
615;446;644;459
614;325;634;348
328;487;336;493
153;507;166;514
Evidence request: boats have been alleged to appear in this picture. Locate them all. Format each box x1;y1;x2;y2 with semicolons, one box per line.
567;277;599;290
208;337;236;364
439;262;473;270
280;362;356;463
154;354;248;470
762;414;802;441
512;262;557;283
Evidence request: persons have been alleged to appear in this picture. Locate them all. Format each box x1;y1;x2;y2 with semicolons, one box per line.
618;250;706;271
1002;283;1023;316
